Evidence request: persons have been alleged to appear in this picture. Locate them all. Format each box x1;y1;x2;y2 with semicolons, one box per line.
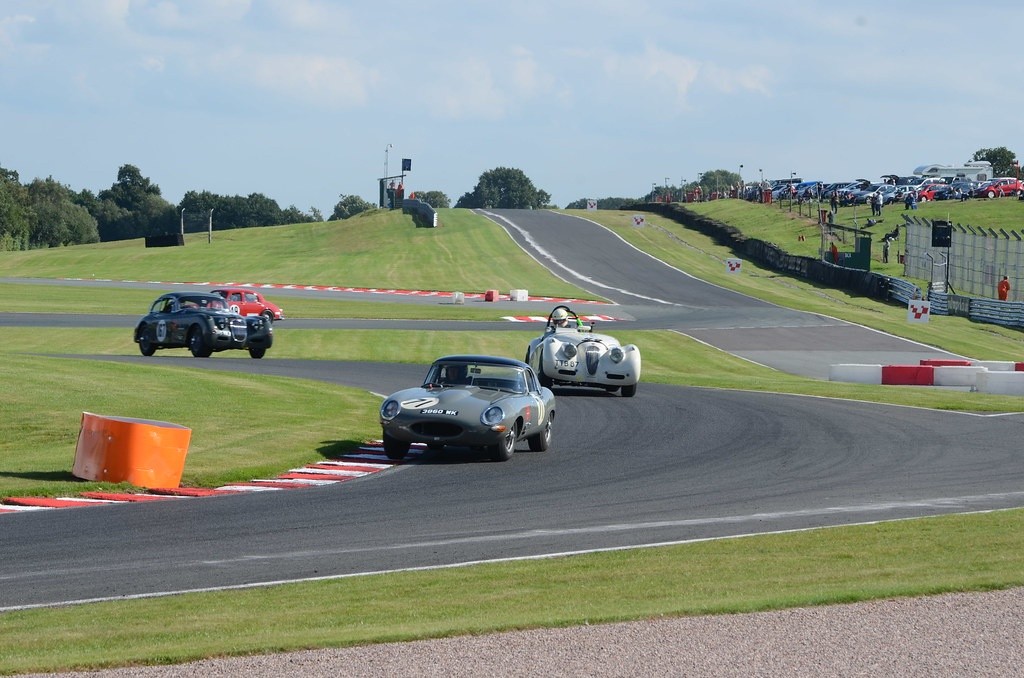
444;365;463;384
389;180;395;189
830;241;839;264
883;239;890;263
397;182;404;197
685;182;1024;229
998;275;1010;301
552;309;572;328
884;223;900;241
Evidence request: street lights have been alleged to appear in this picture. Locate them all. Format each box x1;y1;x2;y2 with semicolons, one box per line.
384;142;393;206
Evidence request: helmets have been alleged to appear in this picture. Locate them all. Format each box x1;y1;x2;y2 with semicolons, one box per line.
552;308;568;325
446;365;468;379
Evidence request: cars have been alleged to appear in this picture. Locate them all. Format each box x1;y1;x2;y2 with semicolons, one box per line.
380;352;561;462
697;175;1024;205
523;306;642;398
130;291;273;361
209;288;283;320
